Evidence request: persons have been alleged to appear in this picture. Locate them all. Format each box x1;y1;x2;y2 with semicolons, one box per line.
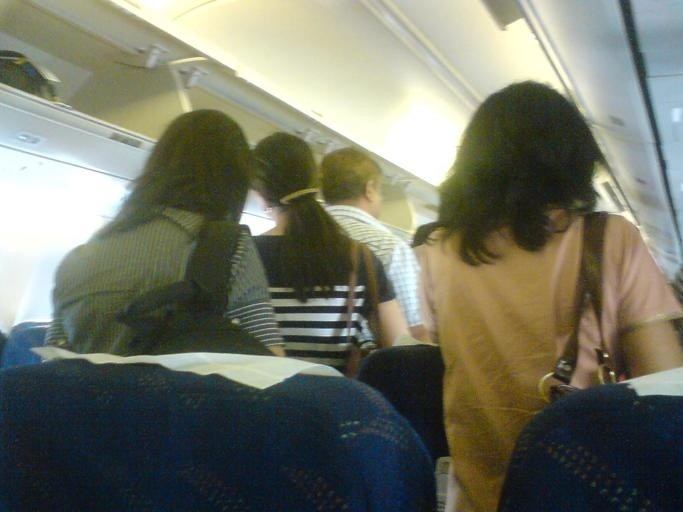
415;77;683;512
243;130;415;377
40;106;289;363
314;142;428;346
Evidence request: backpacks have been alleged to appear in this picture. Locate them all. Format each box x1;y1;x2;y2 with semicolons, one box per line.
114;219;275;355
